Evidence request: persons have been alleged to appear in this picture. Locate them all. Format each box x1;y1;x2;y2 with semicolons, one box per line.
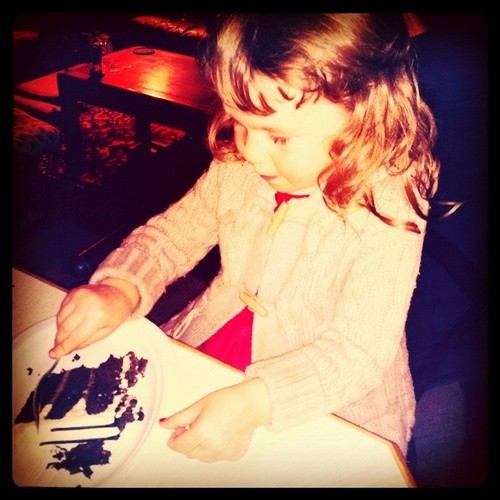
46;11;439;467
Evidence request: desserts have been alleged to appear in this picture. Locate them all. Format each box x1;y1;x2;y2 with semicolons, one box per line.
13;350;147;476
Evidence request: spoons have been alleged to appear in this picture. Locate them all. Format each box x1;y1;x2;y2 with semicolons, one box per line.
33;357;60;431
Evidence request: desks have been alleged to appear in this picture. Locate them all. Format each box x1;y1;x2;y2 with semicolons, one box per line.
57;47;238;187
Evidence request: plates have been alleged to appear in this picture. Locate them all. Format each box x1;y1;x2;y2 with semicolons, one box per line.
13;316;164;490
